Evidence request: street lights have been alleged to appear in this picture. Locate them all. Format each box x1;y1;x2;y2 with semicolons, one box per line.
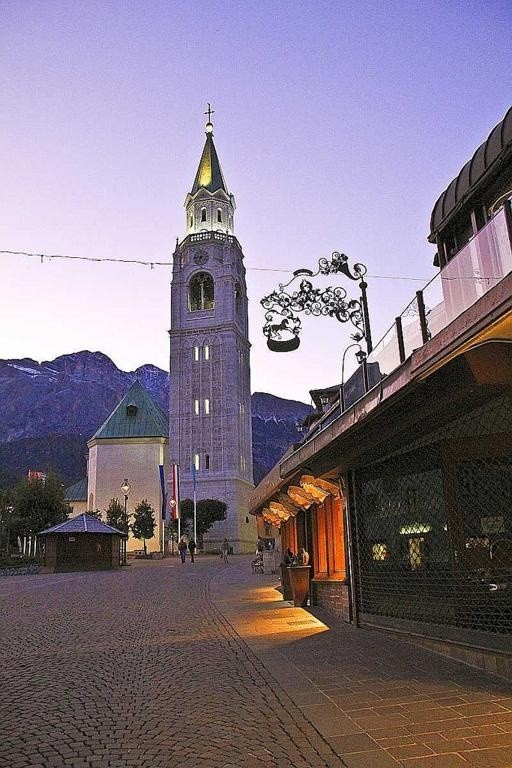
168;495;178;553
117;478;132;566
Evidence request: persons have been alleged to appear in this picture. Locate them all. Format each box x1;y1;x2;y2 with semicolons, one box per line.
188;538;196;563
255;537;273;560
178;539;187;563
220;538;229;564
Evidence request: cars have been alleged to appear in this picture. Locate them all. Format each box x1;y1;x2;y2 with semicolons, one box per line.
259;538;275;550
446;545;511;602
